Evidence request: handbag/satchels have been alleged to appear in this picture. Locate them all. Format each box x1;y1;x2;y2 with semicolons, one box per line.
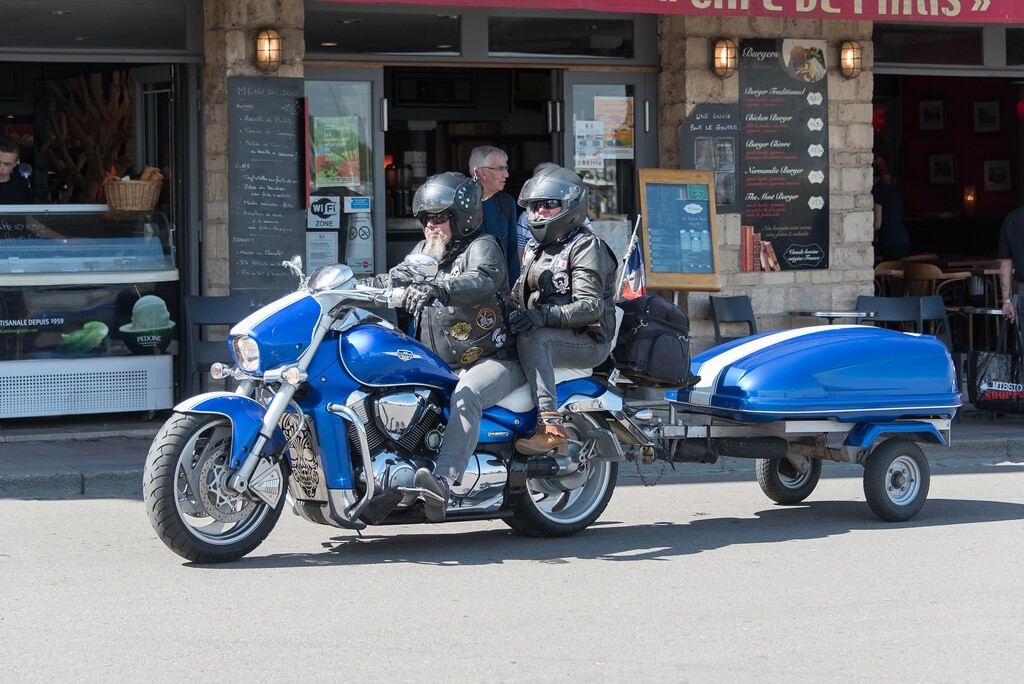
967;315;1024;414
593;294;691;387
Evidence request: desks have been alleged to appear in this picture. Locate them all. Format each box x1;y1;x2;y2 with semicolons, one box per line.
939;267;1016;352
789;309;878;324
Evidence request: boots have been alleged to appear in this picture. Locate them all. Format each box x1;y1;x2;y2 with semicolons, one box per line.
515;412;568;458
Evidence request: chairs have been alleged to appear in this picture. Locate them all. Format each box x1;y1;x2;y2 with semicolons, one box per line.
856;255;974;421
708;295;757;345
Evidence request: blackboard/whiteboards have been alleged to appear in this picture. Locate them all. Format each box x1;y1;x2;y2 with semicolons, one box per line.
679;102;739;213
228;74;306;295
737;36;835;270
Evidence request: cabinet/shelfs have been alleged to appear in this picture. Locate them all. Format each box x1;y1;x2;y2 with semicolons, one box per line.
0;204;180;424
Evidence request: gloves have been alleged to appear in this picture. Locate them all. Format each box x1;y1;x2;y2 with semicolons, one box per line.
512;309;543;336
399;281;444;314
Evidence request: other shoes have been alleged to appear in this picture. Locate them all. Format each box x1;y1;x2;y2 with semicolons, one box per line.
414;468;449;521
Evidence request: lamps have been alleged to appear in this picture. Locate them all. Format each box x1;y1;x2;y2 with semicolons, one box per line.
839;37;863;80
256;29;283;74
713;37;737;80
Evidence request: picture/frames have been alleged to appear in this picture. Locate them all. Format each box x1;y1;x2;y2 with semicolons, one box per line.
918;99;945;132
972;101;999;132
984;161;1011;192
930;153;955;182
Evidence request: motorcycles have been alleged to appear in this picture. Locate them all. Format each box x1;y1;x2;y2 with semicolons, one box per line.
144;255;653;565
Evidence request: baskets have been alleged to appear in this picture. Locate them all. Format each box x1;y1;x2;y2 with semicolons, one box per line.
102;176;162;211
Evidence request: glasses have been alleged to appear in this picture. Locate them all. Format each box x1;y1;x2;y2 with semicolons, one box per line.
418;210;450;225
481;166;509;172
529;200;561;211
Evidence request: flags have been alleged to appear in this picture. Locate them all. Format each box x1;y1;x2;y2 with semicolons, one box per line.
621;243;647;300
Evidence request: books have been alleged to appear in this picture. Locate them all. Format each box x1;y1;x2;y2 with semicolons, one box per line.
741;225;780;273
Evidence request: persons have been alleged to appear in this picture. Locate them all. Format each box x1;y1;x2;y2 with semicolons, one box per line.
356;171;527;522
510;166;618;456
469;145;593;289
874;156;911;261
0;136;33;204
997;208;1024;345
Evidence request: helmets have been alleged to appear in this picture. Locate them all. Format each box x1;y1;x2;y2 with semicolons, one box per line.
519;165;589;246
412;172;484;238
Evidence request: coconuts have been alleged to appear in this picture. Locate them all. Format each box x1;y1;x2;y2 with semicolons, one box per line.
119;295;176;354
